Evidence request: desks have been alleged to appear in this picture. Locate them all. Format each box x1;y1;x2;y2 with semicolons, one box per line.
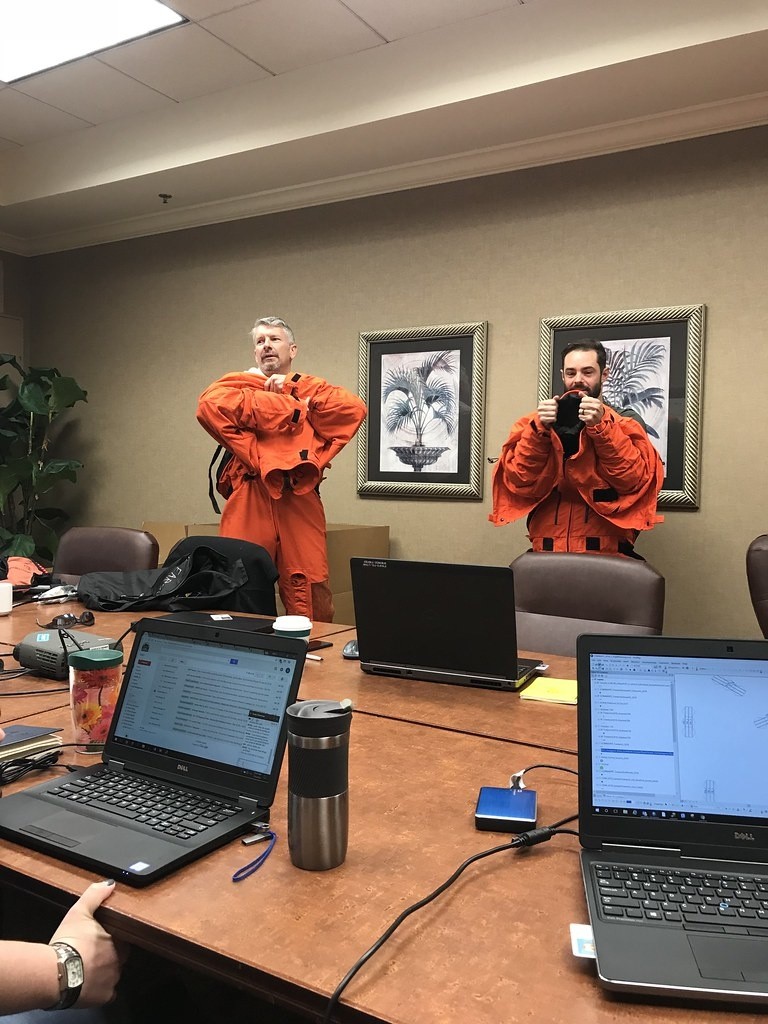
0;595;768;1024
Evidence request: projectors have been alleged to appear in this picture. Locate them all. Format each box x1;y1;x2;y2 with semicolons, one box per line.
13;630;124;680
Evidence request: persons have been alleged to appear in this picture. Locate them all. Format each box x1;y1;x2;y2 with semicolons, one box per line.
197;317;368;623
492;339;664;558
0;879;119;1023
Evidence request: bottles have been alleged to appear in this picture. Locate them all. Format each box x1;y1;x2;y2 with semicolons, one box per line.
69;649;124;755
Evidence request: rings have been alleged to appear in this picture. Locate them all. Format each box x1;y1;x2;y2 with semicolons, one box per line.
582;409;584;415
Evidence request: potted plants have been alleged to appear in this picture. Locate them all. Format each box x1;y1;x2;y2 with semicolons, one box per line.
382;349;456;469
0;351;89;585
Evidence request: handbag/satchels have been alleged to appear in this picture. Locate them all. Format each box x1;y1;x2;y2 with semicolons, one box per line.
77;545;249;612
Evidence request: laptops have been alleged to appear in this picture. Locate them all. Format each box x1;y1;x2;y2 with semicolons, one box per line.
350;556;543;691
0;617;308;883
576;633;768;1003
154;611;276;634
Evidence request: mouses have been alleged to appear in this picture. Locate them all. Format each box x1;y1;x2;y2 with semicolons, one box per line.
342;639;359;659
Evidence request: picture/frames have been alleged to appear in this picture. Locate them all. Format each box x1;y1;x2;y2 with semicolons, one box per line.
537;303;707;512
358;321;489;502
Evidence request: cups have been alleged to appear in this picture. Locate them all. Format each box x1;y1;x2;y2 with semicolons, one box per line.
0;582;13;616
272;614;312;646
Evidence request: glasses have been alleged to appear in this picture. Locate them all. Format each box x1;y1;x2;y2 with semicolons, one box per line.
35;611;94;629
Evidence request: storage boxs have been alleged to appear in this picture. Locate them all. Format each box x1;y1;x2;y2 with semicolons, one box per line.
184;524;390;626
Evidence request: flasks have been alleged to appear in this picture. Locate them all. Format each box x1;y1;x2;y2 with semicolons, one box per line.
285;697;355;872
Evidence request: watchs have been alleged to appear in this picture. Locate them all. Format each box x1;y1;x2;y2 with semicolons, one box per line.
49;942;84;1010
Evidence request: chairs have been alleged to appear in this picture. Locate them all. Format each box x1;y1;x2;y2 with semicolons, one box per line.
745;533;767;641
510;550;666;659
50;524;276;614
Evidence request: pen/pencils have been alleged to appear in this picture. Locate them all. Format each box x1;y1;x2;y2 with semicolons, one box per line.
305;654;323;660
